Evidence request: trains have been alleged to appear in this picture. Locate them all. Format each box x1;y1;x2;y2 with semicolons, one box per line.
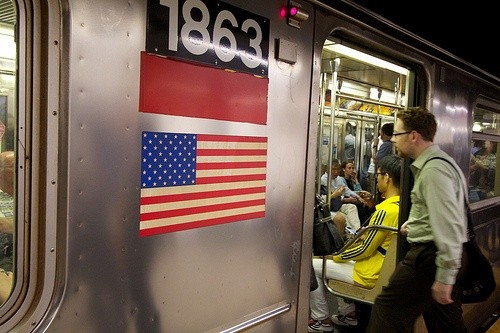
0;2;496;331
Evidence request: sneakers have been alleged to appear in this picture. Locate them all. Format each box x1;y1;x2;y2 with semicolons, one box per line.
332;313;359;327
309;322;334;332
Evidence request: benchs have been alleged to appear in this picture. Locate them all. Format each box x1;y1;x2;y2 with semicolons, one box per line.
322;224;399;333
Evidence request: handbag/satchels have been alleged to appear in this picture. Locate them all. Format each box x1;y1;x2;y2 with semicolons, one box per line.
451;239;497;304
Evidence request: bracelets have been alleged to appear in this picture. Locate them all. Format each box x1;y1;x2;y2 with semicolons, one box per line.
372;144;377;148
355;195;360;198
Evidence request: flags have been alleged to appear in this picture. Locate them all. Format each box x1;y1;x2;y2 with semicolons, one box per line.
140;130;268;237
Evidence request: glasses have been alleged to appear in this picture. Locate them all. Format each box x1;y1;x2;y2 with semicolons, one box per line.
392;131;411;137
374;172;386;178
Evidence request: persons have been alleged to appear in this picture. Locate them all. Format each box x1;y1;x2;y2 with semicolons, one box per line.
320;122;394;241
367;106;469;333
307;154;401;333
470;141;496;199
0;122;14;304
